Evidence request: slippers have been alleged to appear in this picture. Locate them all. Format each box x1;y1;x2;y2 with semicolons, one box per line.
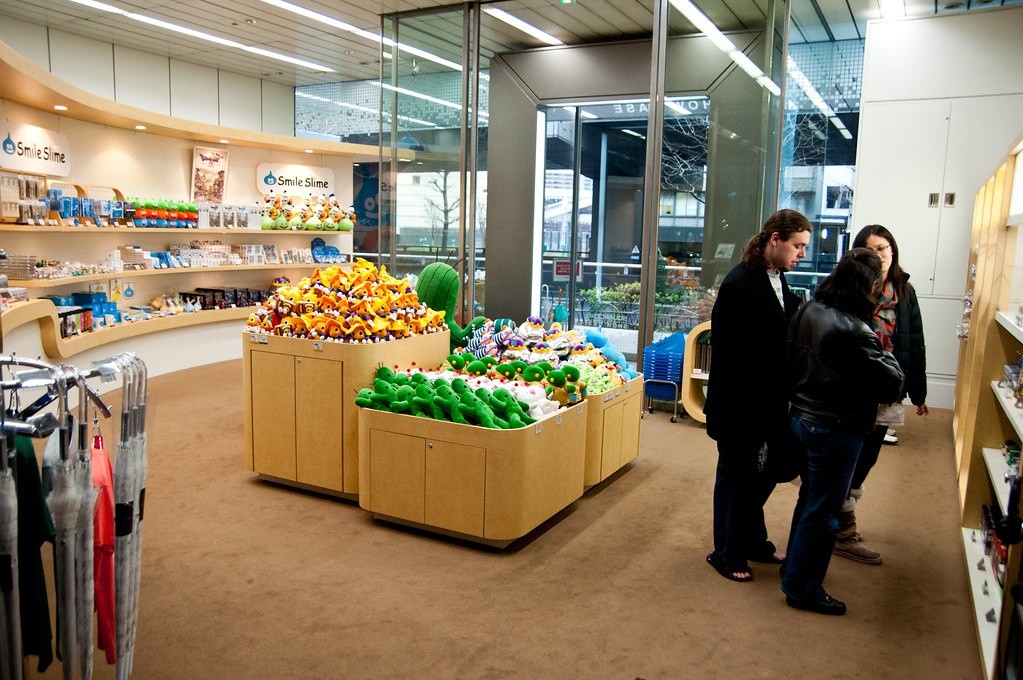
744;541;786;563
707;553;753;582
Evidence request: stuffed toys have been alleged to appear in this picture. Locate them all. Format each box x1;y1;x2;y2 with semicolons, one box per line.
245;257;449;344
126;197;199;228
414;261;486;355
354;316;639;429
261;190;356;231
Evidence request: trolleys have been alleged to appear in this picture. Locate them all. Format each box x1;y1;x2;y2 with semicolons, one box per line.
639;379;686;423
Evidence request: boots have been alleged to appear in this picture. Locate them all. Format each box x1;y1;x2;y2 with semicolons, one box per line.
831;496;883;564
849;484;864;541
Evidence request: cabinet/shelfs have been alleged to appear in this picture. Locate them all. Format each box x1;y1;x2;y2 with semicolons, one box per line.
0;226;356;359
240;325;644;554
952;138;1023;680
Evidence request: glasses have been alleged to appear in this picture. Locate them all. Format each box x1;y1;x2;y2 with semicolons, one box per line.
874;245;890;254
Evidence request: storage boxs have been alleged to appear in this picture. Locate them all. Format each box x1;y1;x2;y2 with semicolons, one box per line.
36;291;129;328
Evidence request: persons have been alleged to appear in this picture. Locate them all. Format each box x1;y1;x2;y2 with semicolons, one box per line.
705;208;804;582
818;226;929;565
780;248;905;616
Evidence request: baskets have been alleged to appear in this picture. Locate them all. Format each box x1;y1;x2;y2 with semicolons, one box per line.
641;329;686;403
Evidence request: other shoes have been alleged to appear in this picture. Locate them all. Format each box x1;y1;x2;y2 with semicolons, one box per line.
883;434;898;444
886;428;896;435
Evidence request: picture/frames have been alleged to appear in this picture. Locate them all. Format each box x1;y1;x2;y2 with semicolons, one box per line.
262;242;281;264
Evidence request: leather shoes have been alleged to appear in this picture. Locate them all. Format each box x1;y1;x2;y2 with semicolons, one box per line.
786;593;846;615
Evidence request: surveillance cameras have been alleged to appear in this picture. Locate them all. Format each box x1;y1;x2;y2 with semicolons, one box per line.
412;65;419;76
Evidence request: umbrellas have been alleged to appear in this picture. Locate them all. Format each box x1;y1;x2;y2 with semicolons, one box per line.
0;353;147;680
